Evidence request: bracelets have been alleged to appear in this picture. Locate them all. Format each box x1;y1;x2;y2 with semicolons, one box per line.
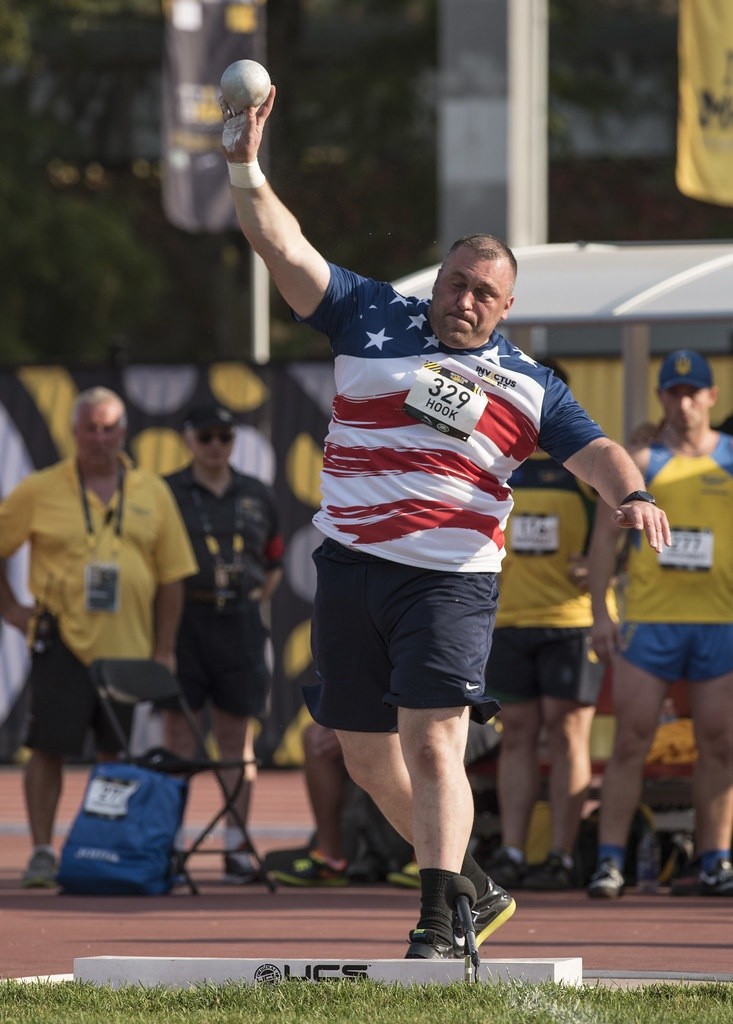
226;160;266;189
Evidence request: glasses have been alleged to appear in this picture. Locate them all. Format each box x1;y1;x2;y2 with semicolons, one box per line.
196;432;232;444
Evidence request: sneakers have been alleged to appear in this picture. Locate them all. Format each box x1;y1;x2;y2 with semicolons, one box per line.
24;849;57;887
527;853;583;888
403;929;454;961
224;854;262;885
451;876;516;959
387;863;421;887
267;850;349;886
698;857;733;895
484;850;527;889
586;857;625;897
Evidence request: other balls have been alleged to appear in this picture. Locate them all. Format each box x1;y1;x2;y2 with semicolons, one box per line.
220;59;271;109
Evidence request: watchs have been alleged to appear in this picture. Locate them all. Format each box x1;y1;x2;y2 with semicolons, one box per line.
620;490;657;507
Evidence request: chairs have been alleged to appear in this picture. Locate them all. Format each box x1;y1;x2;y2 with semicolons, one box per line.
87;657;280;897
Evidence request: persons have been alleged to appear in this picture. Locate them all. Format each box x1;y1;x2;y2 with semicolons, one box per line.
158;401;287;883
265;722;421;888
587;347;733;899
485;353;608;892
218;59;671;959
0;387;199;887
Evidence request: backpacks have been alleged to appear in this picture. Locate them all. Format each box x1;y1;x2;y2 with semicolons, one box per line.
57;747;190;896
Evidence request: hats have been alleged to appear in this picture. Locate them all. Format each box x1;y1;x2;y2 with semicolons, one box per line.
180;403;237;427
659;350;713;389
